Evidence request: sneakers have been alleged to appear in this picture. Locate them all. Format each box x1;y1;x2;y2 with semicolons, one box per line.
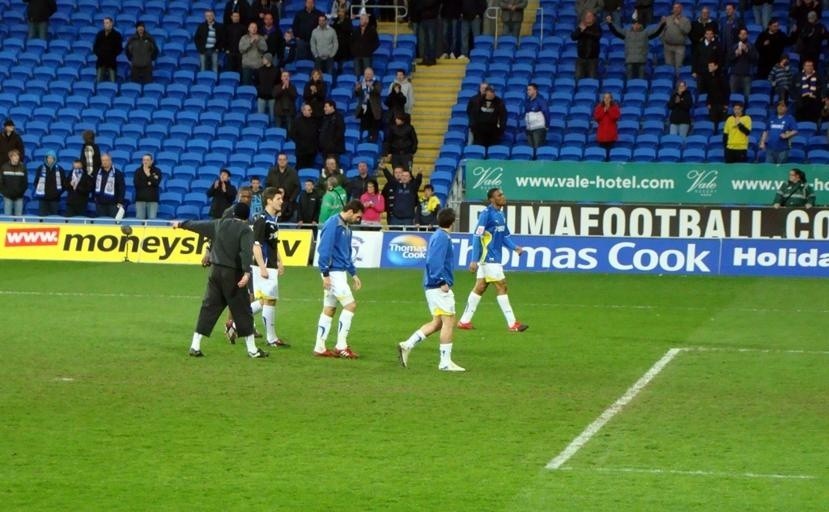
313;348;358;359
225;320;290;358
438;362;465;371
509;323;528;331
457;321;475;329
190;348;204;356
399;342;409;366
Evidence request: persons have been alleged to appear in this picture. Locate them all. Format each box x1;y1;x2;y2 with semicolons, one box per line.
314;199;363;357
458;190;529;333
225;187;288;346
398;208;468;371
203;187;263;335
0;0;829;228
170;203;269;358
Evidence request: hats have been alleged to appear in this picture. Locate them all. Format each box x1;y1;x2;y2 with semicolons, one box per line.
485;85;496;90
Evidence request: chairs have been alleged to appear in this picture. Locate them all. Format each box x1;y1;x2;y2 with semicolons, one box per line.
0;0;829;222
